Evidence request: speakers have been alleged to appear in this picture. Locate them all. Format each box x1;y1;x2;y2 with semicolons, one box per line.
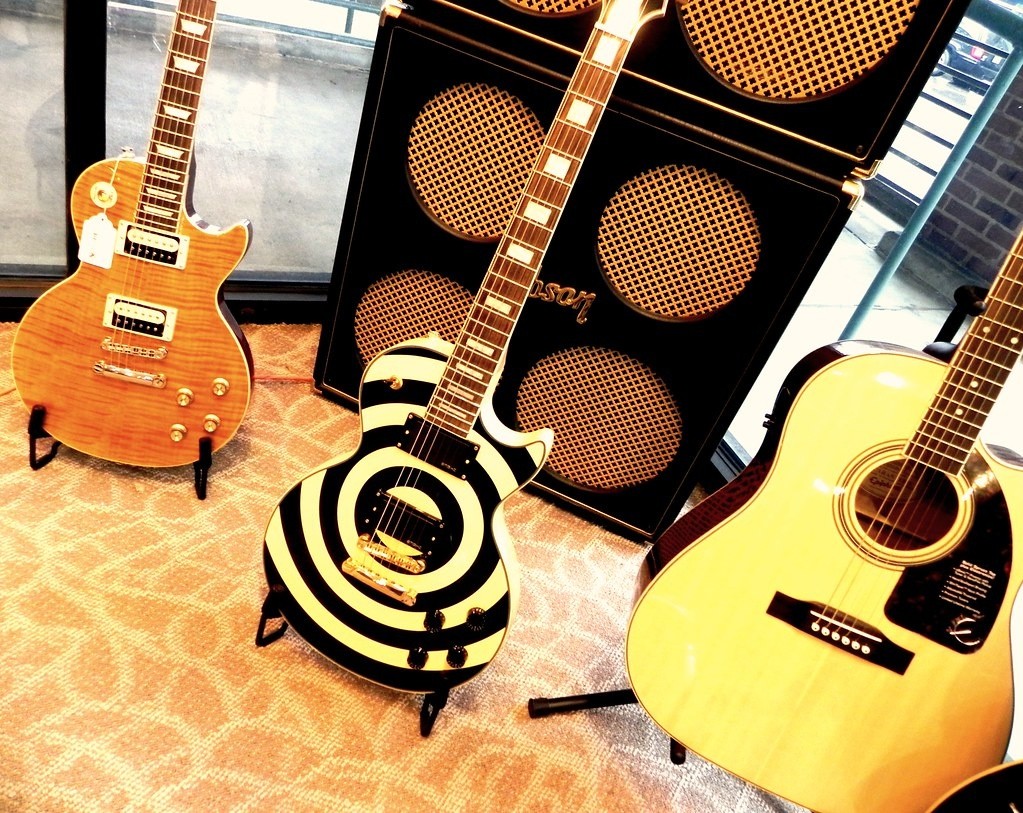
312;0;866;544
386;0;973;184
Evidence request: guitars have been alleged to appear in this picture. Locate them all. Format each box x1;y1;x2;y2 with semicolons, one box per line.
627;234;1023;811
252;2;673;695
10;2;255;472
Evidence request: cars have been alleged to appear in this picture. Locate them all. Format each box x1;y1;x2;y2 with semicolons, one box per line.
931;18;1010;94
